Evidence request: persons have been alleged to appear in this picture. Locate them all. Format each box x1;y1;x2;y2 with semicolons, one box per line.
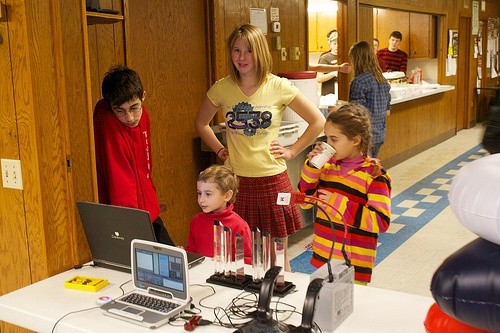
297;104;391;287
193;24;329;274
307;29;409;91
345;41;392;160
93;65;174;246
185;165;252;264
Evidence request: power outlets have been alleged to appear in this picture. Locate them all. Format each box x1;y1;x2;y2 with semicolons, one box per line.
1;158;24;191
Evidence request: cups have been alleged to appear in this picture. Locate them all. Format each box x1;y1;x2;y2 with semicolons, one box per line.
310;142;336;169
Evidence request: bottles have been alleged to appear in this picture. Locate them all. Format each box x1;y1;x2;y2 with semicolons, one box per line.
412;68;422;84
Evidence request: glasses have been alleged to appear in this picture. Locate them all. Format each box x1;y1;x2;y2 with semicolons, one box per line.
113;100;142;117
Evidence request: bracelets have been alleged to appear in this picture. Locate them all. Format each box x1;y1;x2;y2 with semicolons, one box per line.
217;148;224;155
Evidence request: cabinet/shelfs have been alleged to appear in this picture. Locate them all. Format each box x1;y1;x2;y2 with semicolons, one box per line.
372;8;377;39
377;8;410;60
317;0;337;55
308;3;318;55
409;12;437;60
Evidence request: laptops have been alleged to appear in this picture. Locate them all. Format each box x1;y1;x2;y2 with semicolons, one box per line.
75;200;206;274
95;239;191;329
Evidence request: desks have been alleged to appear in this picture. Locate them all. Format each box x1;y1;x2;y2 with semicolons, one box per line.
0;257;437;333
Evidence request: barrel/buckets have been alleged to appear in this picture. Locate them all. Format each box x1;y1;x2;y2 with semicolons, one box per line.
280;78;317;121
